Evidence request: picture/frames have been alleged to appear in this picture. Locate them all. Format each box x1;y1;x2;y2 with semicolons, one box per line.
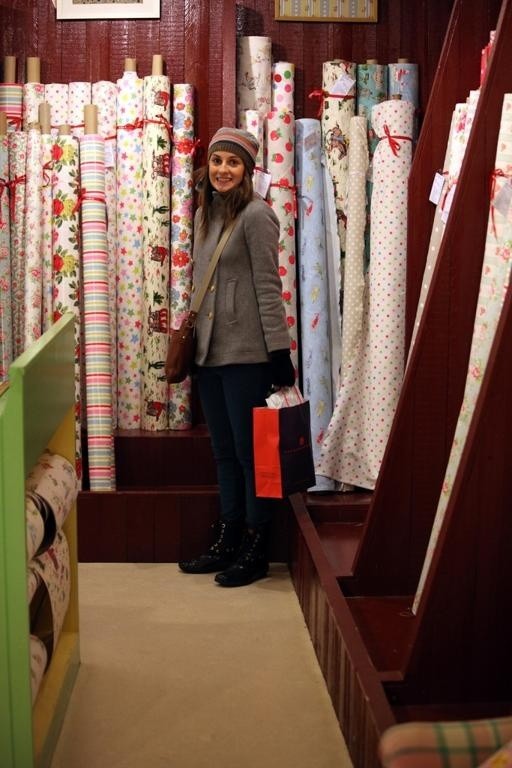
56;0;161;20
274;1;378;23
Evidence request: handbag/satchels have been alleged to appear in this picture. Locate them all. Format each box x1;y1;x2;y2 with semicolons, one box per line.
165;319;197;385
252;401;316;500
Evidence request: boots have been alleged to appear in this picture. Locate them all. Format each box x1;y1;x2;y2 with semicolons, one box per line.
215;528;269;587
179;519;248;573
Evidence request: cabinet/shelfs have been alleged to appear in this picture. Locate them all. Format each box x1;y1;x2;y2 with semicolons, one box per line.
1;312;82;768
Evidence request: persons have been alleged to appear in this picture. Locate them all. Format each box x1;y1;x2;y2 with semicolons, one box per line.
177;126;296;588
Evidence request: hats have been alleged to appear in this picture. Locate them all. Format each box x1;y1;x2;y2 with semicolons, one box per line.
208;127;259;175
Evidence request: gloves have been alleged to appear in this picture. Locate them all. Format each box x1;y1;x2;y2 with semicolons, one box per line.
264;349;295;392
